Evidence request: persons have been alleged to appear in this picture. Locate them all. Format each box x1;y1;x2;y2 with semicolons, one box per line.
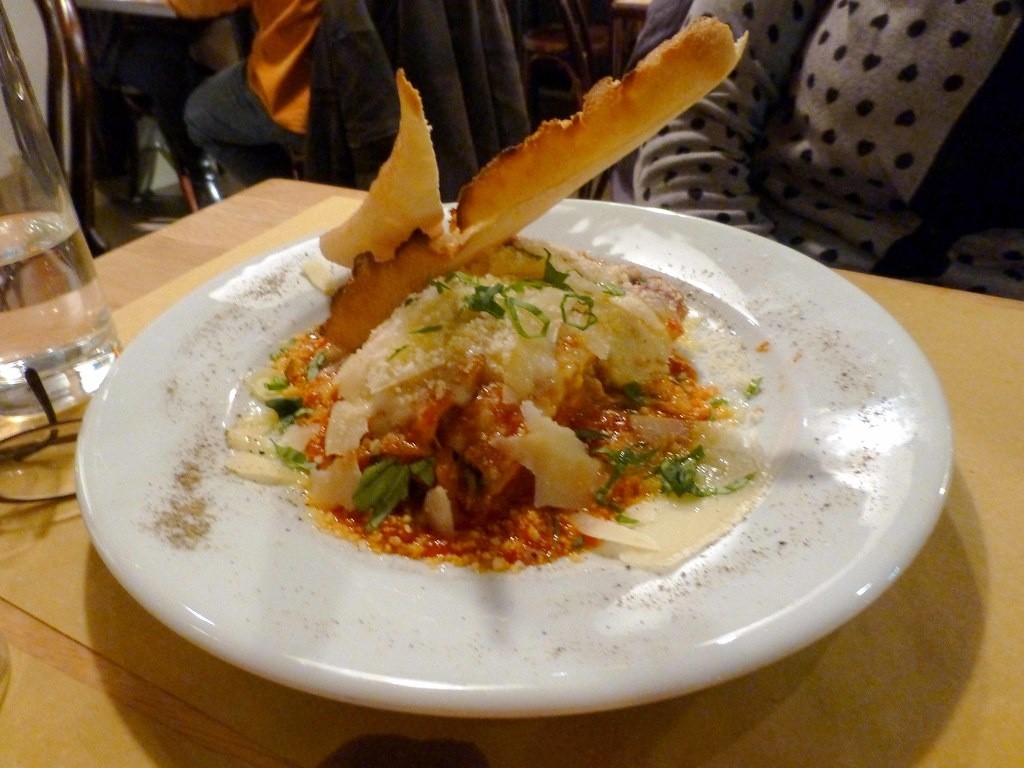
170;0;322;185
613;1;1024;300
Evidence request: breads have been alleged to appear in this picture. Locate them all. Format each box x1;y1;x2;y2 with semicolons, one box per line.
319;16;748;356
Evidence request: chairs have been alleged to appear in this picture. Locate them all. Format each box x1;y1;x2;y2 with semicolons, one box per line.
35;0;655;259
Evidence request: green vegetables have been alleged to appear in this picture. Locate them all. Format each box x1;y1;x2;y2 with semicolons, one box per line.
260;246;756;530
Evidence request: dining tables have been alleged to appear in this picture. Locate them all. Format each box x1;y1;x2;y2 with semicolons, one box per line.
0;178;1024;768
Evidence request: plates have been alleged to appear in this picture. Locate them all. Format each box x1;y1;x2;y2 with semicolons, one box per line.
73;199;948;718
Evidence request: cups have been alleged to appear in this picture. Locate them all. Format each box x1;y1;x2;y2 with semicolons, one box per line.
0;0;115;419
